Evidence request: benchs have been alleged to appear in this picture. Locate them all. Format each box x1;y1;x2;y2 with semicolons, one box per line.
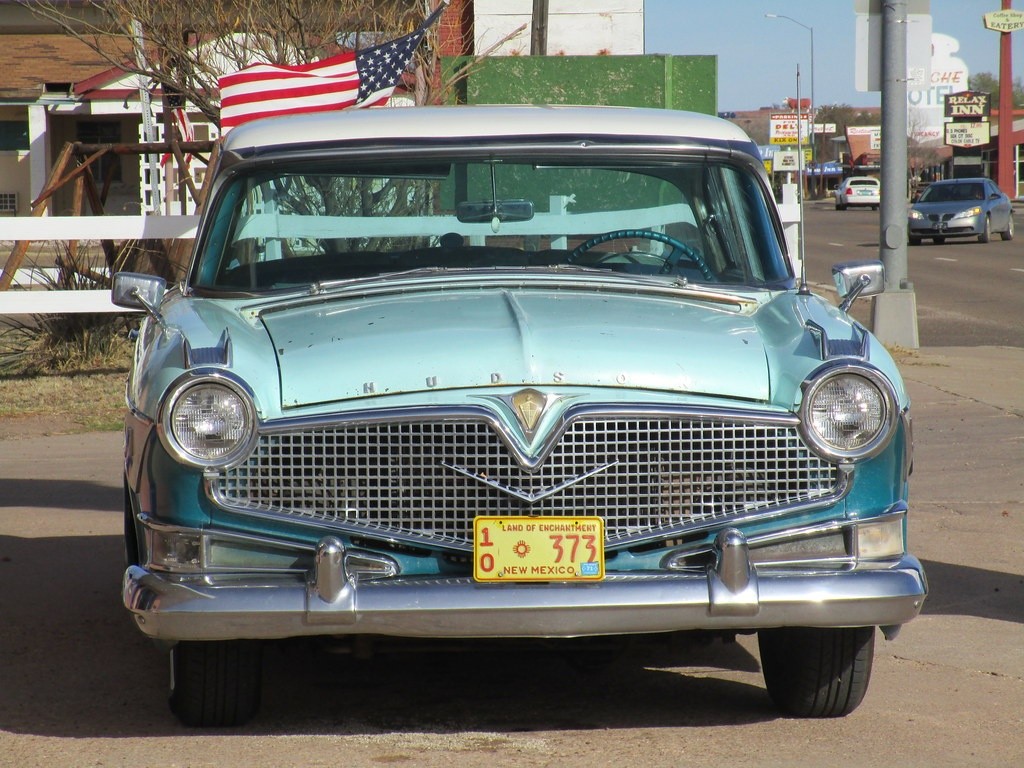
229;247;706;292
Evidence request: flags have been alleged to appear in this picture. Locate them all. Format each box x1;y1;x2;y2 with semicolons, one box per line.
217;0;450;148
160;82;195;168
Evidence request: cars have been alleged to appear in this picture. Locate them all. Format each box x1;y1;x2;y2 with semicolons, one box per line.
908;178;1015;244
111;102;929;729
834;176;880;212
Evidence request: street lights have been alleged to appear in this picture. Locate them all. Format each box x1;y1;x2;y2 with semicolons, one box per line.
762;12;816;201
